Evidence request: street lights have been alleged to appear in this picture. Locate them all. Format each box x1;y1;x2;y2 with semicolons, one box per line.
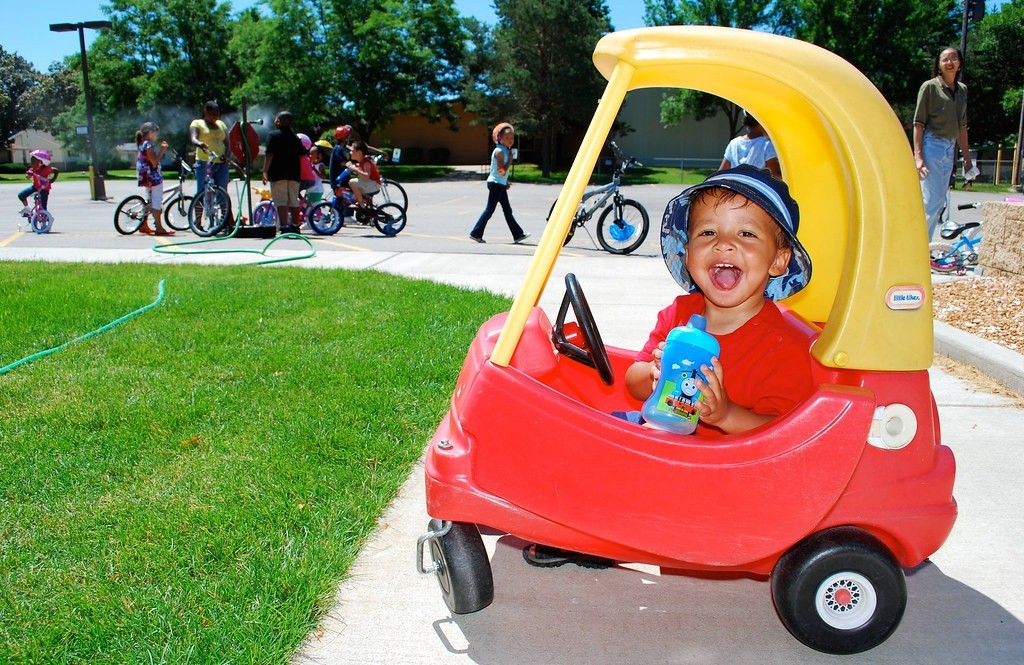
50;20;109;202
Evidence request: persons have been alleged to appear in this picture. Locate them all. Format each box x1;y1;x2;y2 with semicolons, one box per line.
912;45;976;243
136;121;175;236
263;110;389;239
189;101;235;232
17;149;59;233
522;163;813;570
468;122;531;243
718;109;782;181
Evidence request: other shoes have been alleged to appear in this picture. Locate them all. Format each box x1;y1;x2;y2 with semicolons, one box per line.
139;227;155;234
469;235;486;243
156;231;175;235
42;226;49;232
280;227;289;234
348;202;366;210
290;224;300;234
514;233;531;243
19;207;31;214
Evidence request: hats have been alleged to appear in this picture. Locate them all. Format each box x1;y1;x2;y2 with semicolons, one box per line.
659;164;812;302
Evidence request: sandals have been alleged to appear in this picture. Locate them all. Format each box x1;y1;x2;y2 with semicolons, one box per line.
523;542;615;570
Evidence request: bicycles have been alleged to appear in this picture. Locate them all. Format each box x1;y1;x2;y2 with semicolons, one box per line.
929;202;982;272
548;141;650;254
307;181;406;237
113;149;197;235
18;171;53;235
188;144;231;237
253;153;408;235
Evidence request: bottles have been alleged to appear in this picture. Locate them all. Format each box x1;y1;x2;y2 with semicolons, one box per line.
641;315;720;435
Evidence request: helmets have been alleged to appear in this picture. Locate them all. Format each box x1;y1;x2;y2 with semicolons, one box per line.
492;123;514;144
30;150;50;167
334;127;350;140
296;133;312;150
